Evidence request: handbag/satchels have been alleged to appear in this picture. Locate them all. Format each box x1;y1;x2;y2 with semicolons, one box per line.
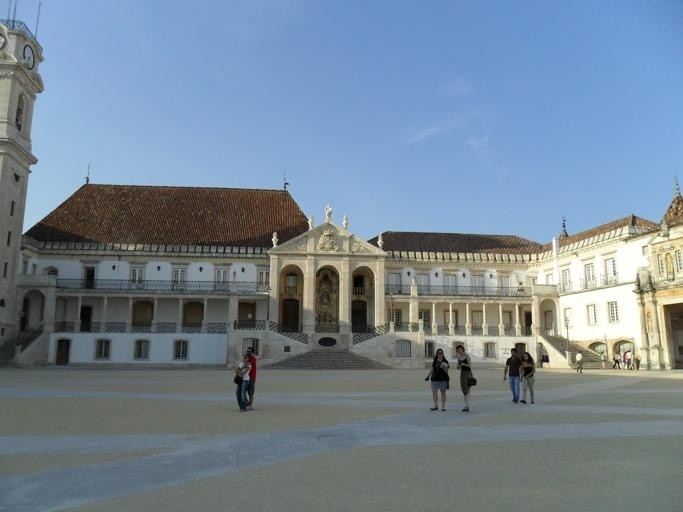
466;377;476;387
234;375;242;384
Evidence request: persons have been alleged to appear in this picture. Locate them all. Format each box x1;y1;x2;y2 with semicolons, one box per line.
504;348;523;403
426;349;449;411
601;351;606;369
455;344;472;412
235;346;256;411
520;352;535;404
575;350;582;376
612;349;640;370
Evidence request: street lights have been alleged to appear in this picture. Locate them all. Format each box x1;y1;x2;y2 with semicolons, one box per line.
389;290;393;323
266;286;271;318
564;316;570;352
16;311;25;345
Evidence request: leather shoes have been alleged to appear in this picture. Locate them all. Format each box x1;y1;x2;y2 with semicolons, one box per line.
462;406;469;412
430;407;447;412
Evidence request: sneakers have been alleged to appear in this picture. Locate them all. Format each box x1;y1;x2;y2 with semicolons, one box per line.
239;403;254;412
512;399;535;405
